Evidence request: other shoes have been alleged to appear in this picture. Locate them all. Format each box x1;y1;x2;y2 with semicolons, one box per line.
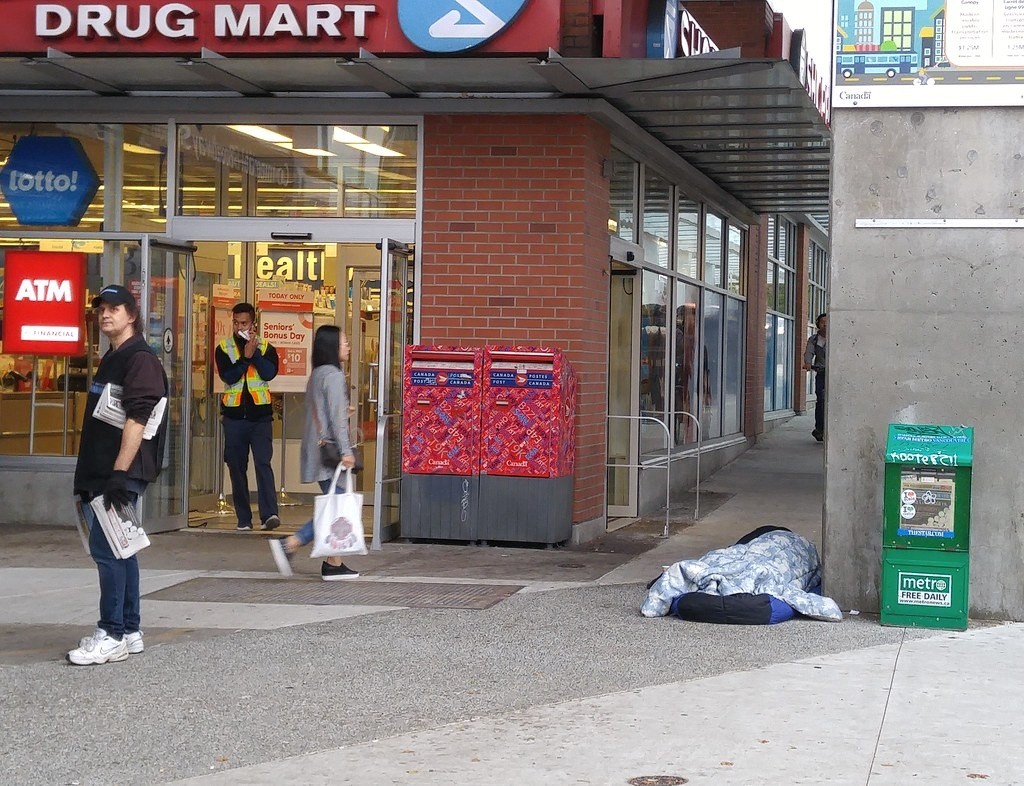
811;430;824;444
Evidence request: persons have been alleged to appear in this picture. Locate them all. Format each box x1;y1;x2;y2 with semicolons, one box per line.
65;285;168;665
804;314;826;442
215;302;280;532
267;325;359;581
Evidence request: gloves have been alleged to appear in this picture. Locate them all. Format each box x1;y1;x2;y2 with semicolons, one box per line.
103;470;133;513
79;491;91;502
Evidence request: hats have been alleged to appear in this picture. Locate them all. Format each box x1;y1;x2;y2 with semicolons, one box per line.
92;285;135;311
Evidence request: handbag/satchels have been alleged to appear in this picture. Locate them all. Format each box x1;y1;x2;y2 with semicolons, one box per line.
309;460;369;558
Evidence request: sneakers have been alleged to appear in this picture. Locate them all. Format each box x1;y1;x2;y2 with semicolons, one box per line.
125;629;144;653
65;628;129;665
236;519;253;531
268;538;294;580
259;515;280;528
321;561;369;581
